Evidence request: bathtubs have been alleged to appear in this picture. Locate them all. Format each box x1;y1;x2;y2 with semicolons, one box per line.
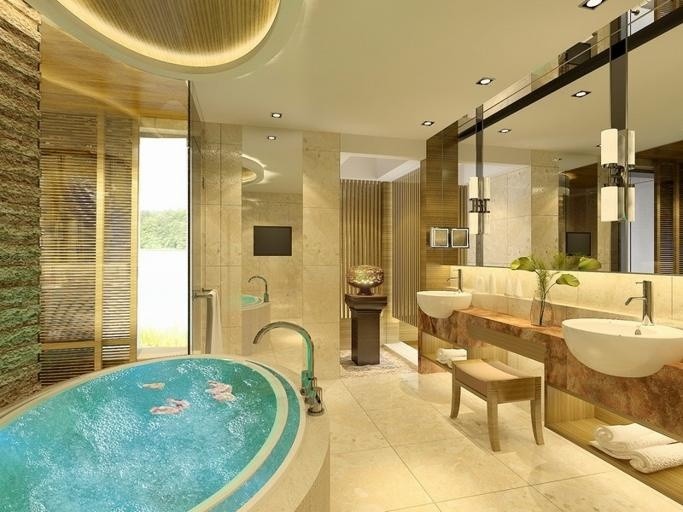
240;295;271;354
0;353;331;512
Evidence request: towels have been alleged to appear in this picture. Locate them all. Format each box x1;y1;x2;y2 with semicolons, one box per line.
608;436;671;455
629;442;683;474
436;347;467;361
447;357;466;368
595;422;653;448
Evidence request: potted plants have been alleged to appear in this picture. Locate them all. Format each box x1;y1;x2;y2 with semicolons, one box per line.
511;255;580;326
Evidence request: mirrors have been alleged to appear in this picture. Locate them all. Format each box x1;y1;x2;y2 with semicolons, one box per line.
612;3;683;275
483;32;612;272
456;105;483;267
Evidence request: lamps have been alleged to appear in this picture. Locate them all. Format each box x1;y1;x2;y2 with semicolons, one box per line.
468;205;479;235
601;128;623;171
600;175;622;223
624;182;636;222
468;176;478;205
625;129;636;171
482;175;491;213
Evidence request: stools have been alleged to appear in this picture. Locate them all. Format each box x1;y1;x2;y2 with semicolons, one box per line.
450;359;544;451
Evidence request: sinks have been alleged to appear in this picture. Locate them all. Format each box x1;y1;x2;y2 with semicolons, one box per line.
417;290;472;318
561;318;683;378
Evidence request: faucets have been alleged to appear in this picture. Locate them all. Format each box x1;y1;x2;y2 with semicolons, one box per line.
622;281;654;326
253;319;325;414
446;269;462;292
247;276;269;302
